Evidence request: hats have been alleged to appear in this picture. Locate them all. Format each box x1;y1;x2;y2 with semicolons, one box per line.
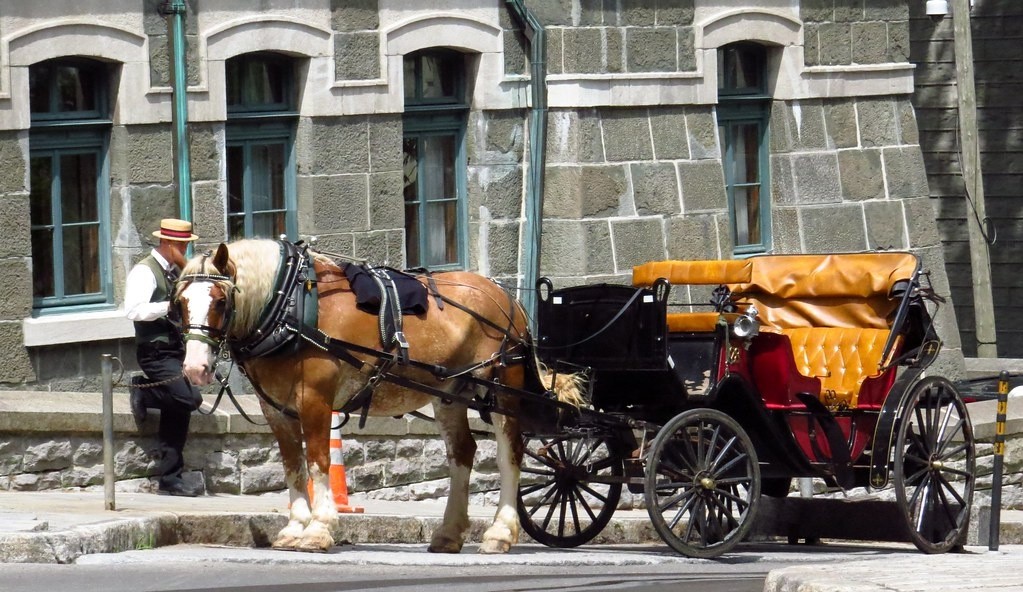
151;218;200;242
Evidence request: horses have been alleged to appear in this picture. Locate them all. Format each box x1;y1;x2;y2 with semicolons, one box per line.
168;238;587;555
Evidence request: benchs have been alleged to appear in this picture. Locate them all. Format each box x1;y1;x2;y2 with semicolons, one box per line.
750;326;903;409
630;259;756;406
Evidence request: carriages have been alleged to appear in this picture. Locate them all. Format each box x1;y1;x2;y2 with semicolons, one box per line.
165;230;981;560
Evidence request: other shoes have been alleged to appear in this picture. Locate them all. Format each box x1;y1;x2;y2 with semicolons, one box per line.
127;376;147;422
157;480;198;497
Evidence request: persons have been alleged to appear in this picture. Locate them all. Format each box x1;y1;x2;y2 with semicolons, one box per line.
125;219;203;496
